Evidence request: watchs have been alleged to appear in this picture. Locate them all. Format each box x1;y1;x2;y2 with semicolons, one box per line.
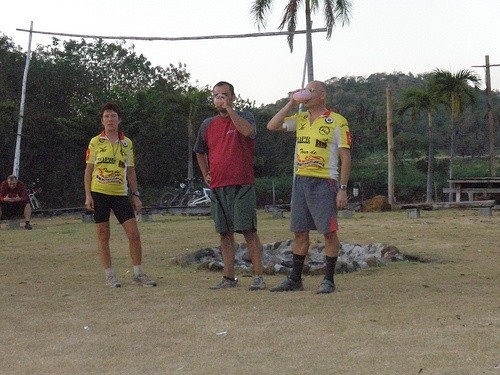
338;183;347;190
131;191;140;196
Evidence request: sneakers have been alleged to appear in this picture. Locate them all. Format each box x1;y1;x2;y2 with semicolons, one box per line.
317;279;336;293
106;273;121;288
209;277;238;289
249;277;266;290
268;279;304;292
132;273;157;287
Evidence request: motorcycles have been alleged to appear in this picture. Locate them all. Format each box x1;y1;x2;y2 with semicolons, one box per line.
166;176;212;217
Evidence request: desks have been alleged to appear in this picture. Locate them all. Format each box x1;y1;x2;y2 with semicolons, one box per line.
447;177;500;210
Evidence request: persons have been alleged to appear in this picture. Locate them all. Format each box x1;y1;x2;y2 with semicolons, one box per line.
0;174;33;229
84;102;159;288
193;81;270;290
266;81;352;296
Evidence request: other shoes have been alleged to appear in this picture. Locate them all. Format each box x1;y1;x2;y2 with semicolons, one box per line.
25;224;32;229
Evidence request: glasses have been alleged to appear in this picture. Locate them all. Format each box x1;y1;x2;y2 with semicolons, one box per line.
309;88;322;94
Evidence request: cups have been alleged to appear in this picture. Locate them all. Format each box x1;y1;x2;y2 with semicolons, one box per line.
214;94;225;106
292;88;312;101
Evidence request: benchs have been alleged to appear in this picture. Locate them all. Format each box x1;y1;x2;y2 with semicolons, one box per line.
402;200;496;219
442;188;500;194
